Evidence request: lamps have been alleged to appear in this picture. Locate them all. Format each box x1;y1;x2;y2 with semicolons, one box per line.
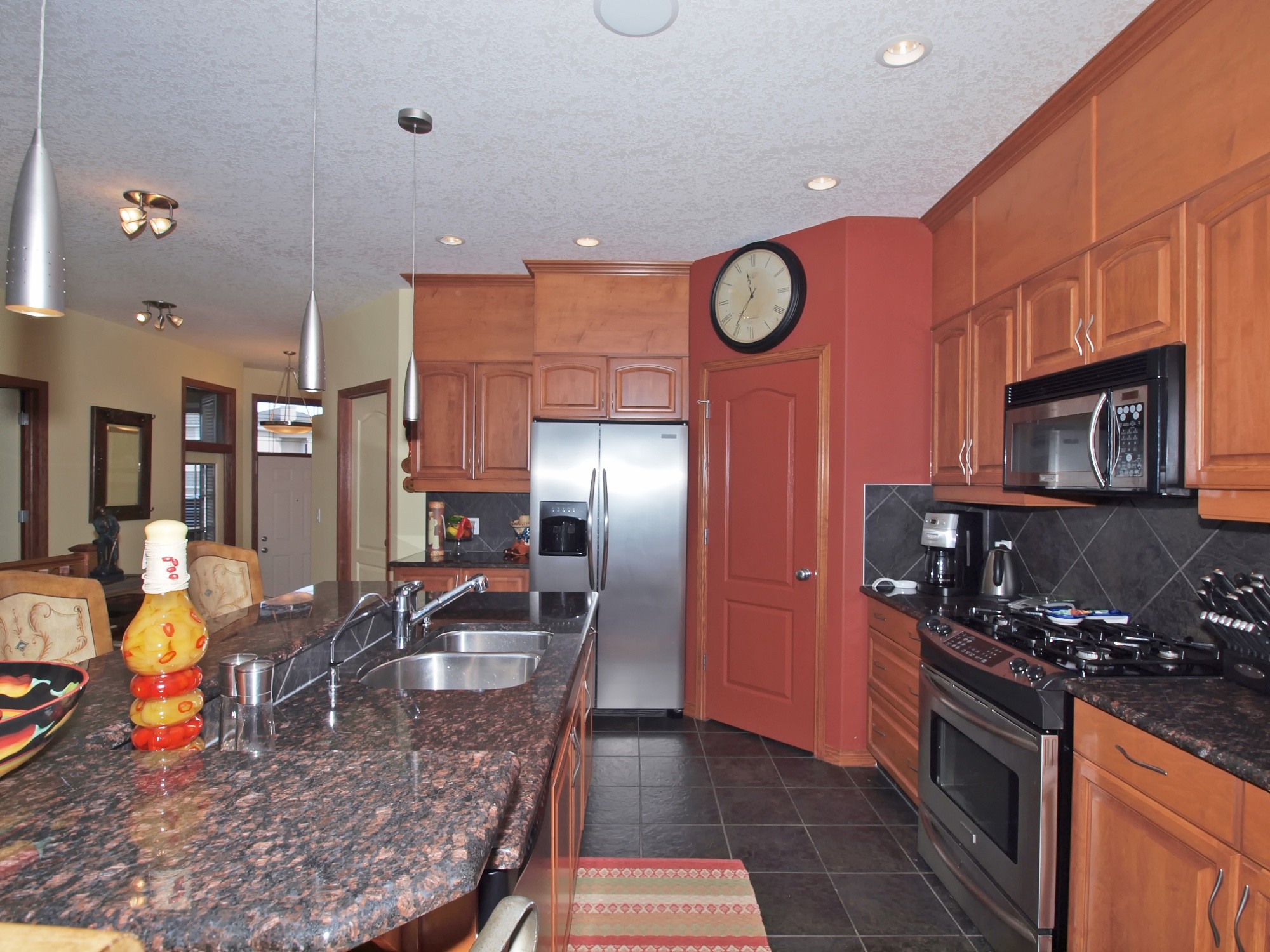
136;300;184;330
259;350;312;436
6;1;69;319
396;108;434;423
118;188;179;242
295;1;328;395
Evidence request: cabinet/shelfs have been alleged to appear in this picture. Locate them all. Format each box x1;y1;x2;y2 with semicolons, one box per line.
548;635;596;951
528;351;690;423
931;286;1016;486
402;361;538;496
1189;159;1270;524
861;579;923;812
1057;698;1270;952
1019;200;1186;387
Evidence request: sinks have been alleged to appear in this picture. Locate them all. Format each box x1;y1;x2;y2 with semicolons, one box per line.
411;629;553;657
353;652;543;692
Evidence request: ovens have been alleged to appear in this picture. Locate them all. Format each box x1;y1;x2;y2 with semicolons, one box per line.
918;661;1060;928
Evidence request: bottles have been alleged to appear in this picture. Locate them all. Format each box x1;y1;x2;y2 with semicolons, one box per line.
217;654;276;752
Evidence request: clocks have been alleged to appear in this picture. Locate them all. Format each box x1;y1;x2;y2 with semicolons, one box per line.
709;241;808;354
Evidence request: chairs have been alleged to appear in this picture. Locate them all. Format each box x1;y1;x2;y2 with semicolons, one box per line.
0;567;115;666
186;541;265;623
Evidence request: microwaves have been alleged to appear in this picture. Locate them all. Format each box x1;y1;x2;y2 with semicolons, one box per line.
1002;344;1198;496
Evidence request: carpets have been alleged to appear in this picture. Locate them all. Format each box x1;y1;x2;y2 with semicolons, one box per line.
567;858;770;951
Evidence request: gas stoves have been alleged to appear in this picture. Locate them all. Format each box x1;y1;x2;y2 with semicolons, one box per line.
917;603;1223;729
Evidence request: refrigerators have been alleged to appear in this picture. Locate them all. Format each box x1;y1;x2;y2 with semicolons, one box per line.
530;415;686;718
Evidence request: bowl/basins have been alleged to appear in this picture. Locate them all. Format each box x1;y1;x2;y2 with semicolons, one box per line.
1045;608;1129;626
0;659;90;777
445;521;475;553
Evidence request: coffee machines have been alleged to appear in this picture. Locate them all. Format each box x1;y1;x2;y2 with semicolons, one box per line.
916;509;983;595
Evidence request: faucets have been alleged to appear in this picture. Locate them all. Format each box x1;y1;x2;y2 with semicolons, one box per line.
391;573;489;644
328;592;391;667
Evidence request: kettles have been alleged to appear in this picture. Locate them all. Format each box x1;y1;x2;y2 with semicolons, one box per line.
978;544;1022;602
